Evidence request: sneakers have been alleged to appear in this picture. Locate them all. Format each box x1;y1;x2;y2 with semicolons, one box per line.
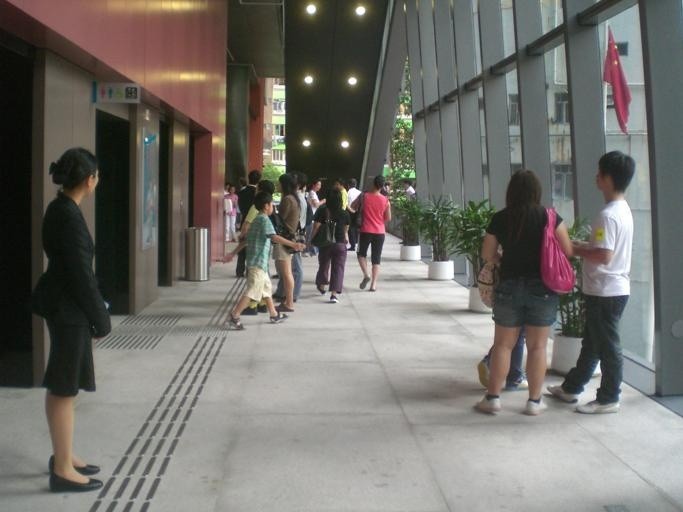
476;358;618;415
229;276;371;330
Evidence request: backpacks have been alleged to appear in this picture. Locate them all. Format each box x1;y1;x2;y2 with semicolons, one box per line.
476;244;503;307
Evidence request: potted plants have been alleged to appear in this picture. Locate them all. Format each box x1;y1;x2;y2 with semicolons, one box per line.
447;198;495;314
391;191;422;260
419;193;458;281
551;215;593;377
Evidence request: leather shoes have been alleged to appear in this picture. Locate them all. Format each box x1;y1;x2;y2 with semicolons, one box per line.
49;472;102;491
49;456;99;475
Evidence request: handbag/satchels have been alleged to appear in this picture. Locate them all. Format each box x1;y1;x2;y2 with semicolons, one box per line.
283;228;304;253
354;212;361;227
269;205;289;243
311;219;336;247
540;208;575;294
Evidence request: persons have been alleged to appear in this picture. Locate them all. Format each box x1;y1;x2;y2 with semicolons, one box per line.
546;152;636;413
29;147;111;492
474;168;572;416
478;324;530;392
402;179;417;201
222;169;391;330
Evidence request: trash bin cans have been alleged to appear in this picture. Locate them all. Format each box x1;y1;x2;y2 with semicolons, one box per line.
184;227;209;281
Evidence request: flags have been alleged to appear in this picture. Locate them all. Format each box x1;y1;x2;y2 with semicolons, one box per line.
602;24;633;135
